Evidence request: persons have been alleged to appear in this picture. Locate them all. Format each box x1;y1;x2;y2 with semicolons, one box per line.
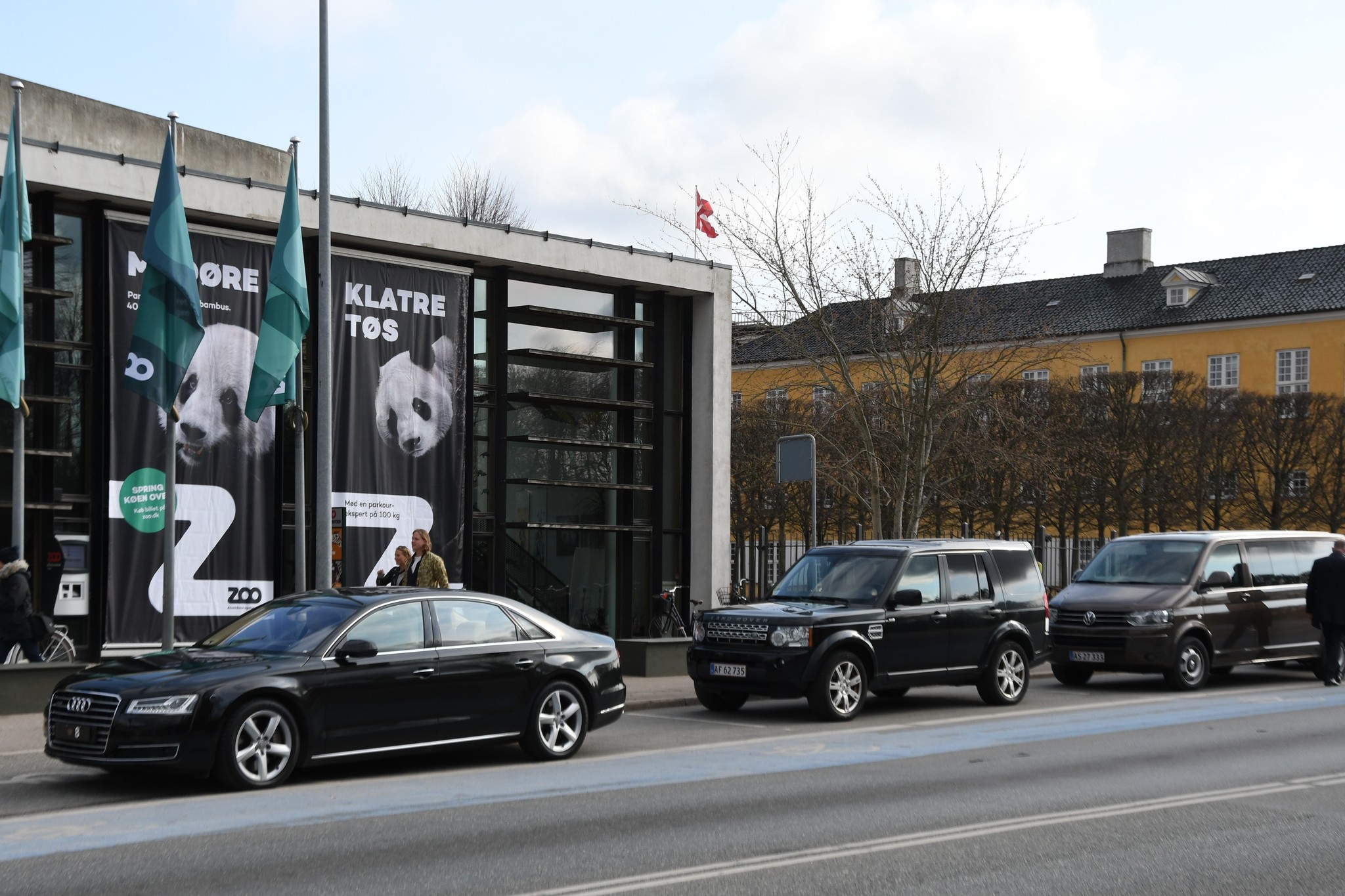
1306;539;1345;687
1216;563;1278;660
402;529;449;589
0;547;44;665
376;545;411;586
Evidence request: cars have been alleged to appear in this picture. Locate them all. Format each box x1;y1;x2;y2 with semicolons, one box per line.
40;586;631;794
1040;527;1345;688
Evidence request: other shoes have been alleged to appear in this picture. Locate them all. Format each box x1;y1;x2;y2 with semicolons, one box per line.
1326;680;1341;687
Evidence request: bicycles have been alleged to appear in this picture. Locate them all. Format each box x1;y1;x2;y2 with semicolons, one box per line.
646;582;703;637
713;578;752;606
6;621;80;663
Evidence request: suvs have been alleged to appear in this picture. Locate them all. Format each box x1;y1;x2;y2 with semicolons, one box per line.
684;537;1059;719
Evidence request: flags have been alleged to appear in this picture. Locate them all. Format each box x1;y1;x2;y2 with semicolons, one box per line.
244;152;310;423
122;126;205;415
697;190;719;238
0;105;33;408
